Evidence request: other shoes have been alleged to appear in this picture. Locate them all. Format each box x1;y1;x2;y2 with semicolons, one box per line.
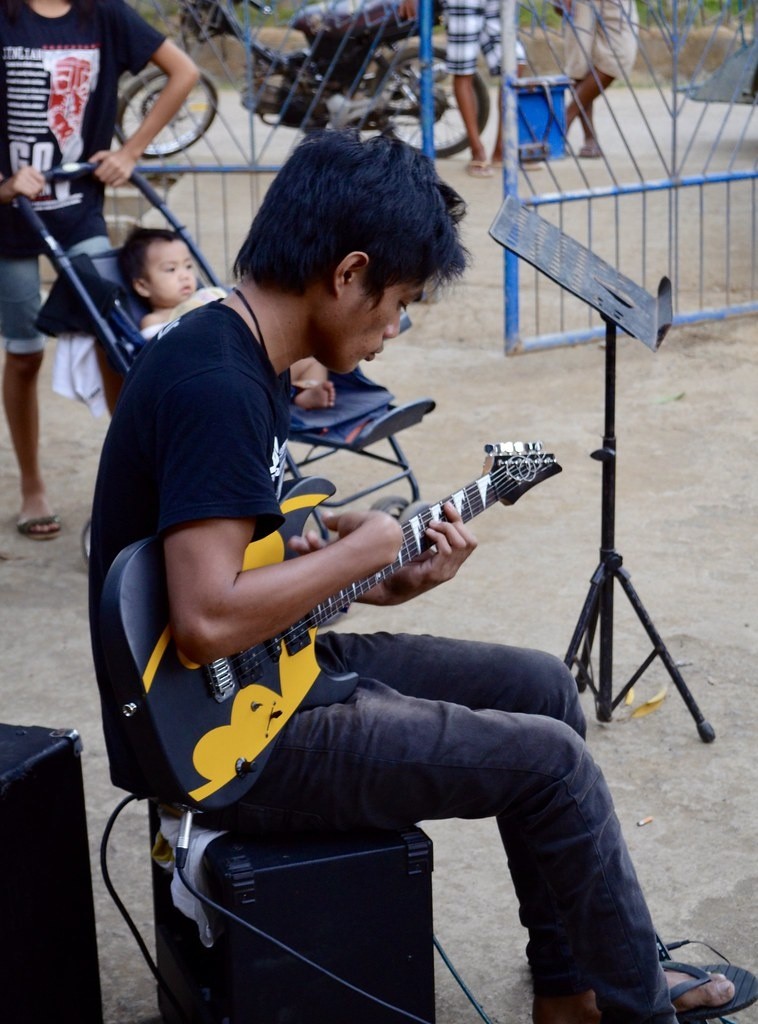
580;145;601;157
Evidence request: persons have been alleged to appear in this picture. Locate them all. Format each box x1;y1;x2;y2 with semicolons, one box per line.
0;0;202;543
552;0;639;157
118;229;337;409
88;128;758;1024
445;0;542;175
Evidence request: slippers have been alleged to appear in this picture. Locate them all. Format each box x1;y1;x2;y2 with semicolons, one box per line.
17;516;63;540
659;960;758;1020
469;160;493;178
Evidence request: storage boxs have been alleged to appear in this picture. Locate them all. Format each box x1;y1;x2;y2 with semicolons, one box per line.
514;75;575;160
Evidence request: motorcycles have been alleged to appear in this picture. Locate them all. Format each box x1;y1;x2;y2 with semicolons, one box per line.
111;0;492;160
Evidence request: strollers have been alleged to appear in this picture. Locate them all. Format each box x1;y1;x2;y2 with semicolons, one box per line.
16;162;437;542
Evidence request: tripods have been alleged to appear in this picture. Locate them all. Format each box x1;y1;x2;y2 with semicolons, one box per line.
486;196;716;743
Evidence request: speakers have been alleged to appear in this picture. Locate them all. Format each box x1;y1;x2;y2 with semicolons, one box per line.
0;723;104;1024
149;800;436;1024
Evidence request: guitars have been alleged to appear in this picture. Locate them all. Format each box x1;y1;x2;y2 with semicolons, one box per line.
103;441;563;810
